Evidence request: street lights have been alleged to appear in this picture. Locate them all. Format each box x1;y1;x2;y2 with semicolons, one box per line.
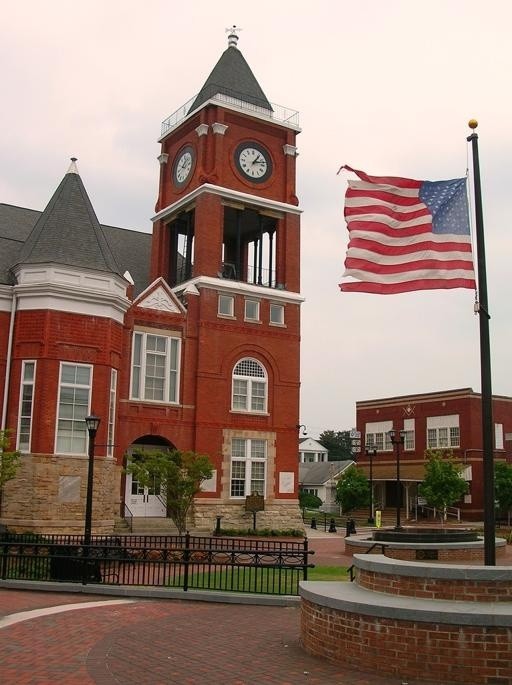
389;429;405;529
83;409;102;553
363;445;378;524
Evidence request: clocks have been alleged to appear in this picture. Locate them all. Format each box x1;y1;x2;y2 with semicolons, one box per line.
234;142;273;183
172;145;195;188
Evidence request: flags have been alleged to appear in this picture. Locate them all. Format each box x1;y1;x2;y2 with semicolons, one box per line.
337;164;477;294
